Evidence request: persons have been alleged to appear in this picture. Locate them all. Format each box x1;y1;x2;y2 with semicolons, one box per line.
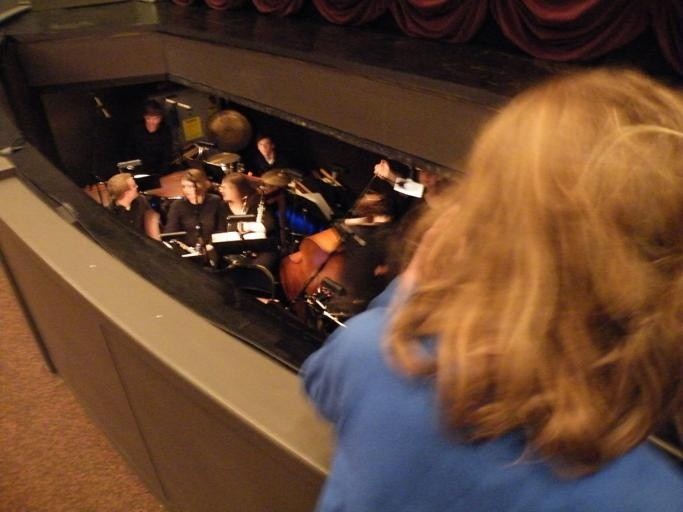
299;65;683;512
